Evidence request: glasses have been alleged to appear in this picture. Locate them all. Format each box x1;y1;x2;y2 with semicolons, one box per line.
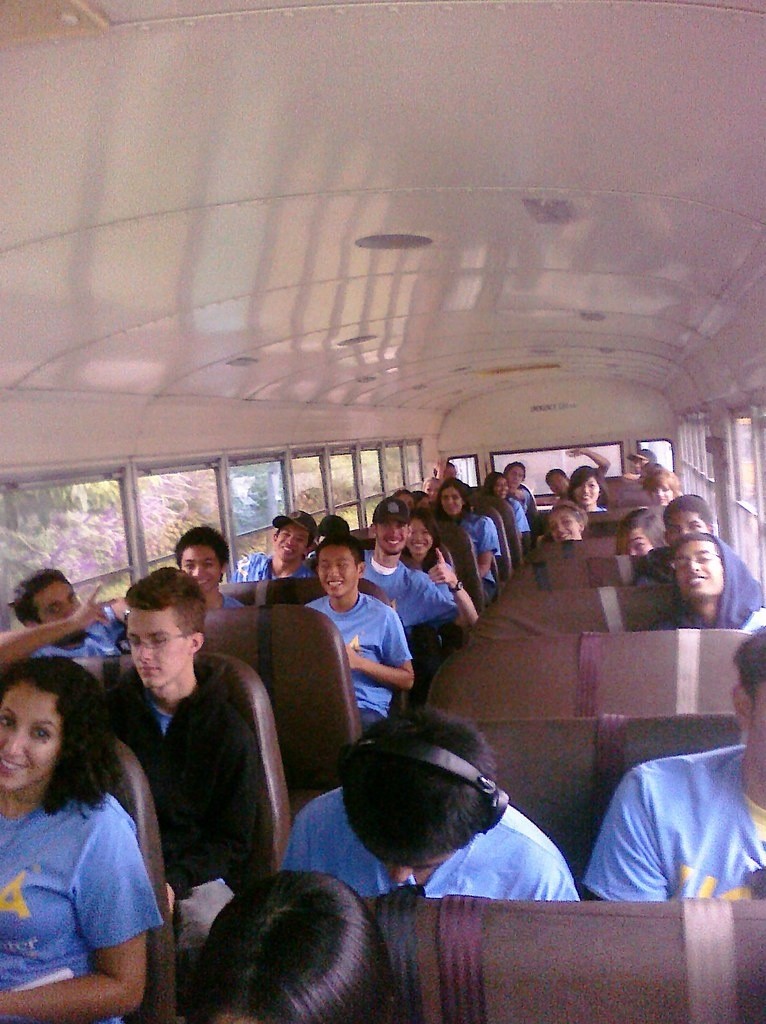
127;629;189;650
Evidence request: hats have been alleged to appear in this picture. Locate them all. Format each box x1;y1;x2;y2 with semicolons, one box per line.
626;449;658;464
273;509;318;540
371;497;412;527
317;514;351;538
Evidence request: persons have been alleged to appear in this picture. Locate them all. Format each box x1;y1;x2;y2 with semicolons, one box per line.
280;703;580;902
581;630;766;902
0;657;165;1024
176;449;766;695
105;567;274;1000
187;870;408;1024
0;569;131;677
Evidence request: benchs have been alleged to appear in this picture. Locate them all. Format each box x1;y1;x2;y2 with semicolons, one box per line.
68;477;766;1024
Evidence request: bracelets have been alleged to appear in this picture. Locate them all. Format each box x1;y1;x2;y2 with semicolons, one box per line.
124;610;129;621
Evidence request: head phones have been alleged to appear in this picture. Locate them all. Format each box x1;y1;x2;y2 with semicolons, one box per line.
335;731;508;835
